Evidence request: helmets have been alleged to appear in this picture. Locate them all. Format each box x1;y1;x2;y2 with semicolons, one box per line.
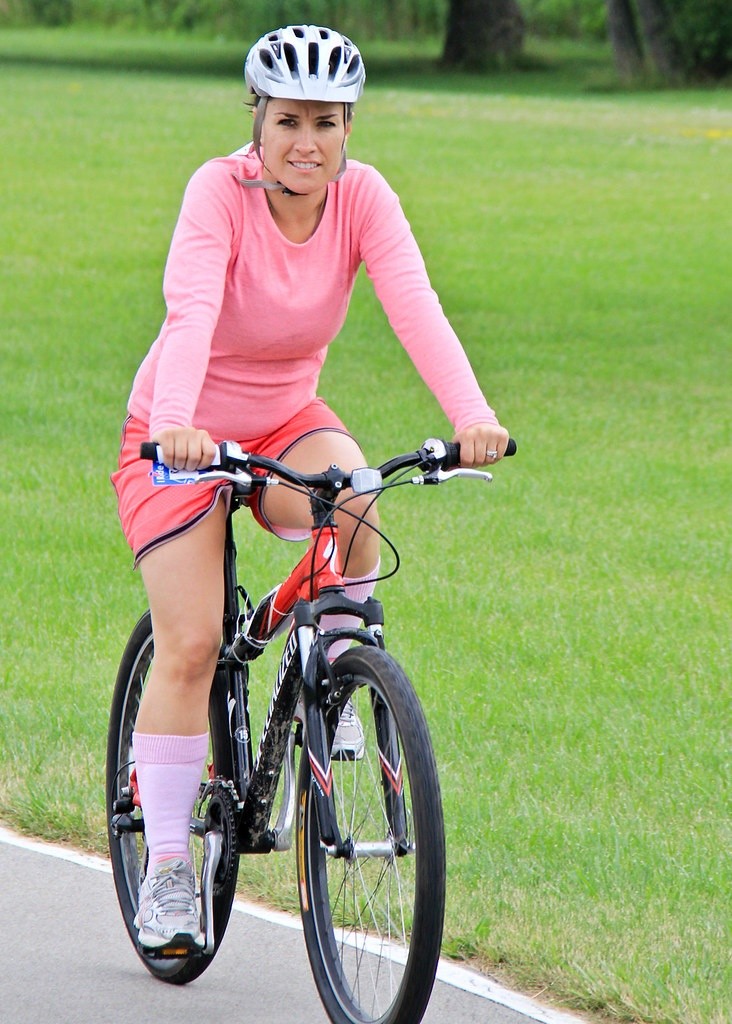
244;24;367;102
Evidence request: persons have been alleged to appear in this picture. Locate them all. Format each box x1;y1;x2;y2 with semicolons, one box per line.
109;22;507;953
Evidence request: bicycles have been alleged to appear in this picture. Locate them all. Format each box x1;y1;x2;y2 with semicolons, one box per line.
102;435;448;1024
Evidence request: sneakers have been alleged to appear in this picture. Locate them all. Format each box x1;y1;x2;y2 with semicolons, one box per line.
133;856;206;952
330;696;366;762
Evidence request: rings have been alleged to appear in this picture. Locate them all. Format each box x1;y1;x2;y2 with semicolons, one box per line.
487;450;498;458
